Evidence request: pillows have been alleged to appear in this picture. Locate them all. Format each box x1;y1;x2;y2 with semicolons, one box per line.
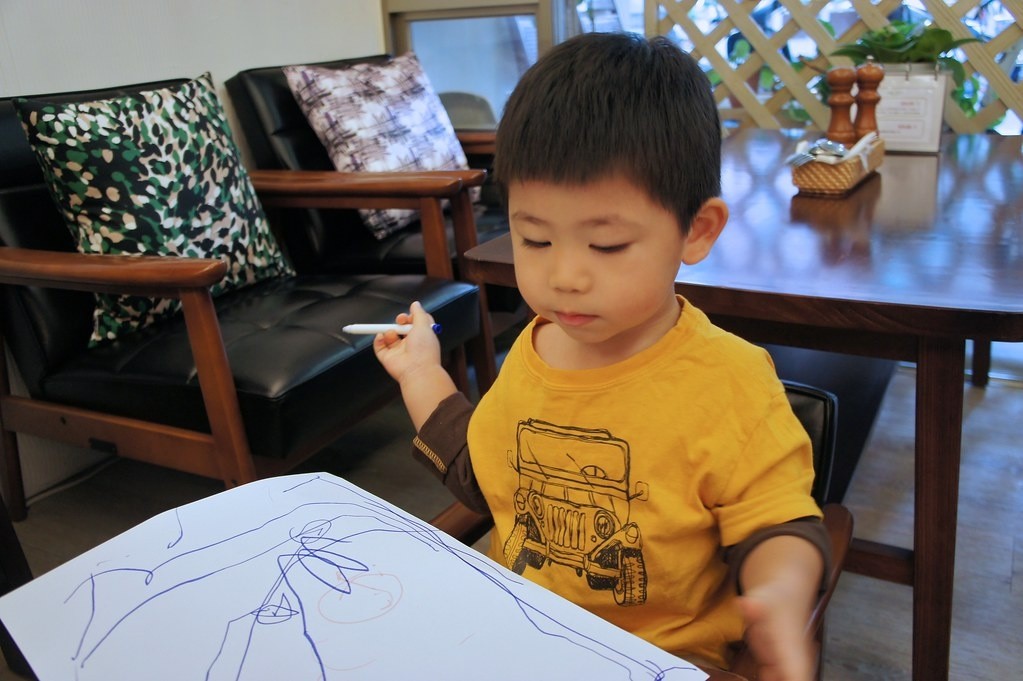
11;70;297;354
279;52;483;242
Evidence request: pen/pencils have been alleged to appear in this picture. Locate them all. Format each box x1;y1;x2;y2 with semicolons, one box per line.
342;323;442;335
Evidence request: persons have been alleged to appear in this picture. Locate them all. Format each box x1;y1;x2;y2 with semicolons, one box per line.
372;30;833;681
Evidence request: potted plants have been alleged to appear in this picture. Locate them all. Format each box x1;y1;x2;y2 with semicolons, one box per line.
700;40;778;107
830;18;989;154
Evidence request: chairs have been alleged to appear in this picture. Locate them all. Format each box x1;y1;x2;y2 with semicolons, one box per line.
429;379;854;681
224;52;536;401
0;78;474;524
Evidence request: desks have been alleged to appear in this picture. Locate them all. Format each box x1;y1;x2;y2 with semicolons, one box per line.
462;123;1023;681
